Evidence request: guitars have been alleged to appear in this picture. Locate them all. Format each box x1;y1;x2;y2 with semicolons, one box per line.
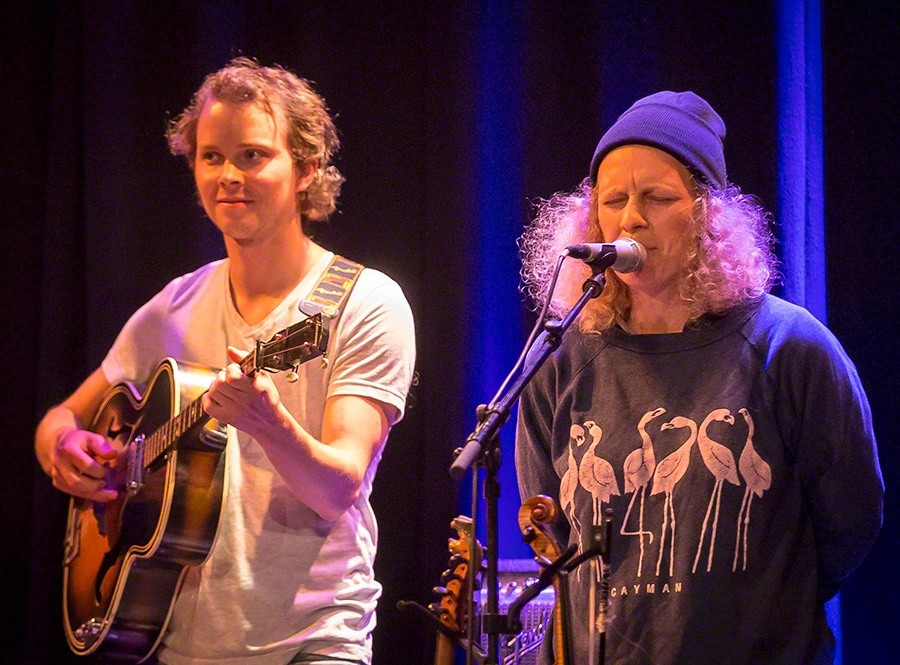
430;515;487;665
60;313;330;665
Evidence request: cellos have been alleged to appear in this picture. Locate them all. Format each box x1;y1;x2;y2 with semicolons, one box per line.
516;494;573;665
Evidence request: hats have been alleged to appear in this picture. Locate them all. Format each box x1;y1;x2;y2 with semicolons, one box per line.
590;90;727;195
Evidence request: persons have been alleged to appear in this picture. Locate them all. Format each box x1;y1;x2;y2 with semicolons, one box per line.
36;56;416;665
515;90;884;665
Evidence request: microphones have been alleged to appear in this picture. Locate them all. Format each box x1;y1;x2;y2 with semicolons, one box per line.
567;238;650;274
